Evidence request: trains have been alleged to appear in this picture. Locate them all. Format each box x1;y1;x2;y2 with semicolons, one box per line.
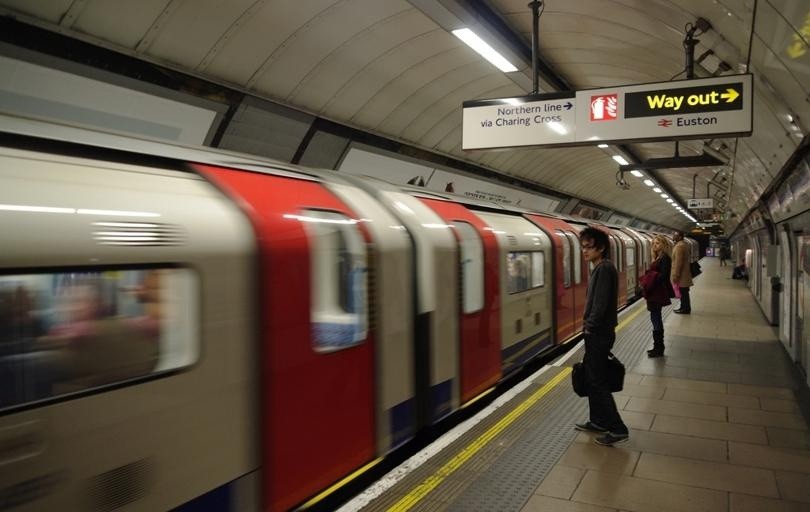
0;110;700;511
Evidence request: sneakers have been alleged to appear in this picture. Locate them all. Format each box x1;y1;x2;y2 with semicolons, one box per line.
575;420;609;432
673;307;691;314
594;430;629;446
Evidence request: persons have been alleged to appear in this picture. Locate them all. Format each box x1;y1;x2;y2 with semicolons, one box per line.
718;242;727;266
733;259;745;278
575;228;628;445
638;234;676;357
671;231;693;313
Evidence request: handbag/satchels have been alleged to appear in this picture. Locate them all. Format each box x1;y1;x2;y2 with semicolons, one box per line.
690;260;702;278
570;350;626;398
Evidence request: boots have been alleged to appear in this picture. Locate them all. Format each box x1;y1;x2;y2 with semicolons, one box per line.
647;328;665;357
